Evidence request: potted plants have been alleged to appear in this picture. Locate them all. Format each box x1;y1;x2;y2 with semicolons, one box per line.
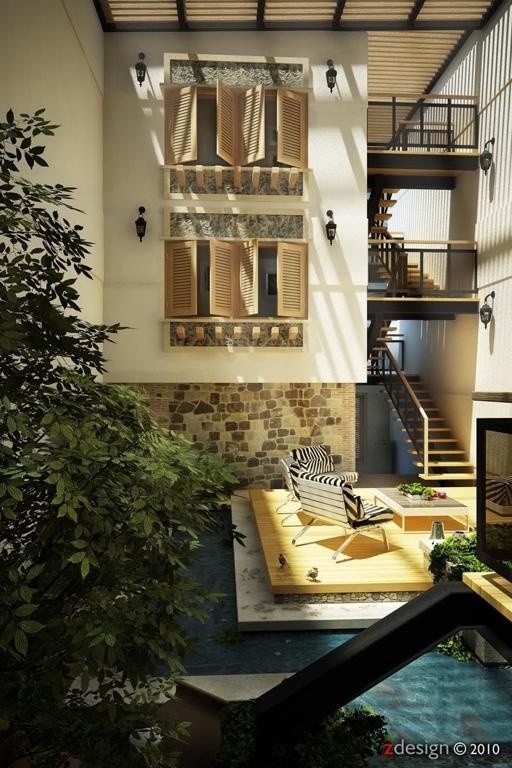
397;484;405;495
405;482;430;500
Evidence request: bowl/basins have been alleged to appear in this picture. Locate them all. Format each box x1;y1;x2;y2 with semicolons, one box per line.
438;492;446;498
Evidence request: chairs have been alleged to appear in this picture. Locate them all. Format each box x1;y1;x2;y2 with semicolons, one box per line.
485;475;512;516
279;443;394;561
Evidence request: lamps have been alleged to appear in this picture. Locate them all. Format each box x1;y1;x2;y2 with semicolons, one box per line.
326;59;337;93
135;206;147;243
325;210;337;245
479;137;495;176
135;52;146;87
480;291;495;329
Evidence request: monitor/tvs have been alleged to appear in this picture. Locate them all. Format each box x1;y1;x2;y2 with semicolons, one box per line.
476;418;512;583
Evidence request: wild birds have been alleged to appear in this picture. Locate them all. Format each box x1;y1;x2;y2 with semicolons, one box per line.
278;553;291;569
304;566;319;583
